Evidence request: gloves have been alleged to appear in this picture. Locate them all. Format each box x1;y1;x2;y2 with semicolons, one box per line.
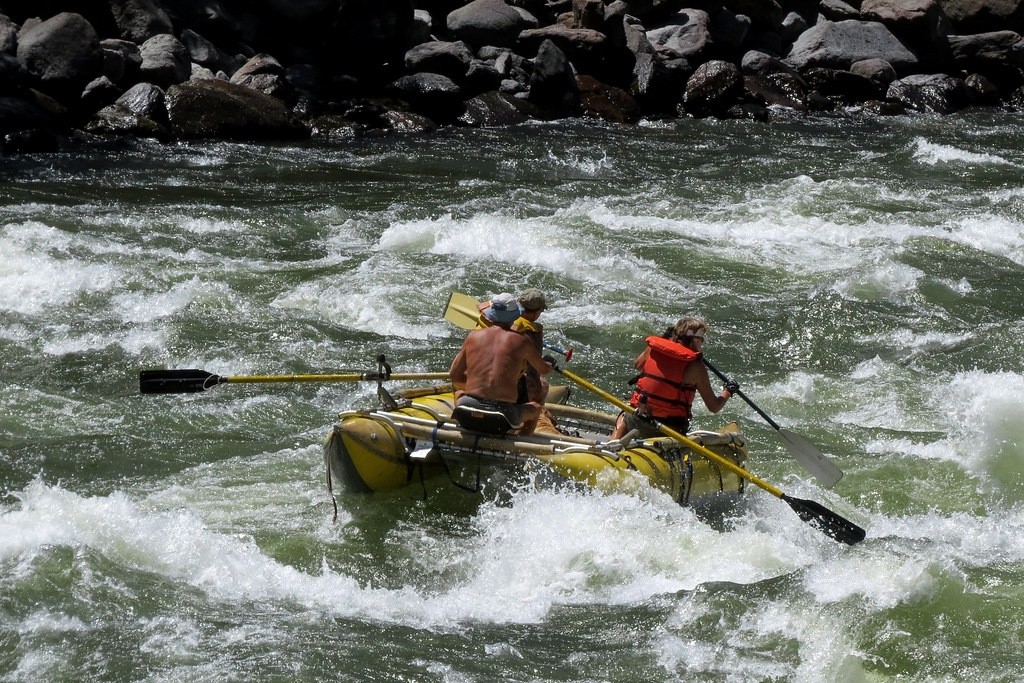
544;355;557;369
663;326;674;339
723;378;740;397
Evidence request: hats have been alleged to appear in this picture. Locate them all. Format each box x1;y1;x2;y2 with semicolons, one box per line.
519;287;548;312
487;292;520;323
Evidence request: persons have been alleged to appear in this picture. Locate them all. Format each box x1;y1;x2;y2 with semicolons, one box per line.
611;316;740;441
448;288;557;435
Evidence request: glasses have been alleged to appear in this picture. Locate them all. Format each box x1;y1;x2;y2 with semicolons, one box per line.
680;335;704;343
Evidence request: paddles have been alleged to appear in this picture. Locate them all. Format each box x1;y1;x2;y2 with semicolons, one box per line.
139;369;468;394
542;355;867;548
663;322;843;491
442;292;574;363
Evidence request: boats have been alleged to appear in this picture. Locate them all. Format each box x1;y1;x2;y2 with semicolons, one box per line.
320;379;752;518
